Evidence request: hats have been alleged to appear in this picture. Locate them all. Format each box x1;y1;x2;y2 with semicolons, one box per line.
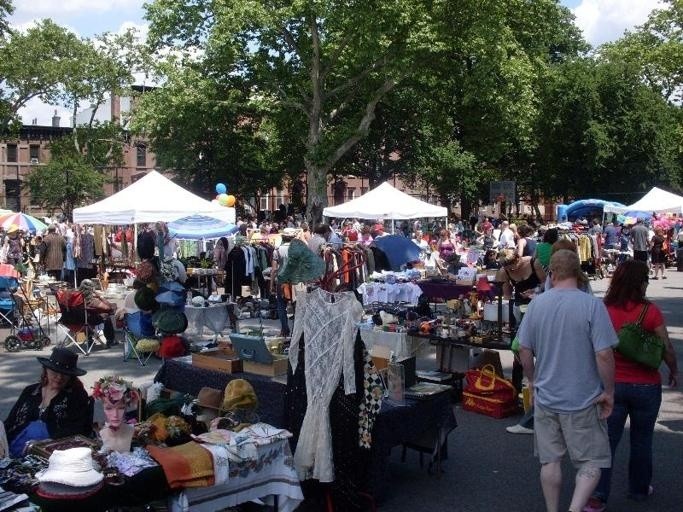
193;386;224;411
278;239;324;284
36;447;106;500
222;378;257;412
37;348;87;376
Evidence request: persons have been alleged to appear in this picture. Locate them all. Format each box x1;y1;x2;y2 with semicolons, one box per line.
0;211;426;337
4;348;88;444
426;211;683;321
518;249;619;510
583;261;679;510
507;239;593;432
93;401;141;452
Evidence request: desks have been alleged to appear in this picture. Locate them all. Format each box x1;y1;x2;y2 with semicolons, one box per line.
1;258;536;512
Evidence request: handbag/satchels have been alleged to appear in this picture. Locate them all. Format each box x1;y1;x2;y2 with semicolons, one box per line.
462;364;518;418
615;321;664;369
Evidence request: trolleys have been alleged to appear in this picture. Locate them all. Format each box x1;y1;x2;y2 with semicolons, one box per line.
3;276;51;351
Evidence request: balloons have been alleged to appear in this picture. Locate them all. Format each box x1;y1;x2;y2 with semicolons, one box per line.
211;183;235;205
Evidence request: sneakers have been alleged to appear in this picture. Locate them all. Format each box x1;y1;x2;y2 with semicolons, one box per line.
107;338;119;347
506;424;534;434
646;485;654;497
583;496;607;512
